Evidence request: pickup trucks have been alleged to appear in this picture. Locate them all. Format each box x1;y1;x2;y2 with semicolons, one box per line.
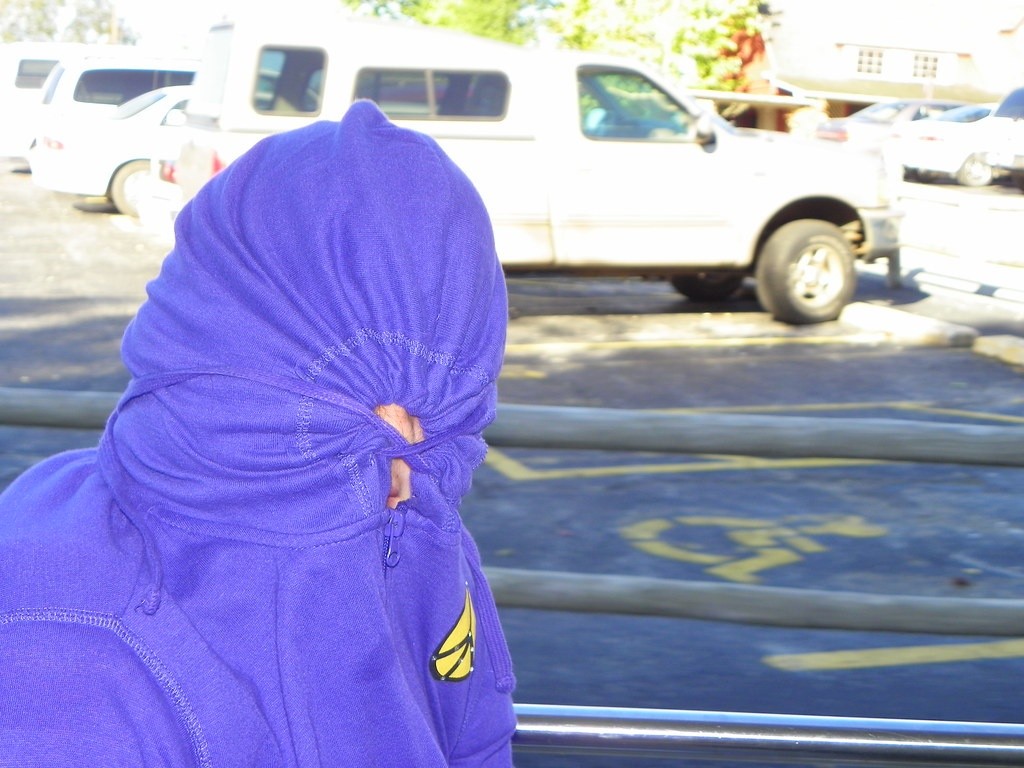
138;6;909;328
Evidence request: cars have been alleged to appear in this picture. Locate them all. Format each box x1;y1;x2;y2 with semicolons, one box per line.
0;35;321;219
809;84;1024;190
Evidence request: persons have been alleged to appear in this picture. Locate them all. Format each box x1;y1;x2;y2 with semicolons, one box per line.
0;97;519;768
723;27;780;131
